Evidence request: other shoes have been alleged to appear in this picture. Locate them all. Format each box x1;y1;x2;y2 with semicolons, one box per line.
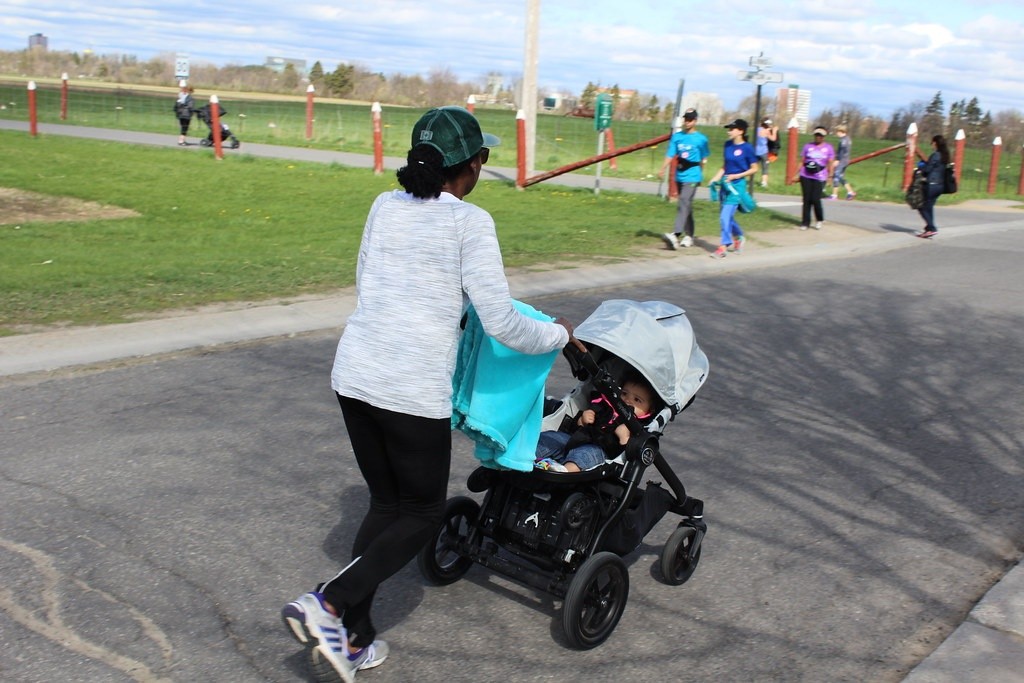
180;142;186;146
827;194;838;200
920;229;938;237
800;225;808;230
845;194;855;201
816;221;825;229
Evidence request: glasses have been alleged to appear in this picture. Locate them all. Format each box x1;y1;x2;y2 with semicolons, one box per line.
930;142;935;145
474;147;490;164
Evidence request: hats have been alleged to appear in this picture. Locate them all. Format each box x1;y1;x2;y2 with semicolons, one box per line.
811;129;827;136
683;109;698;120
724;119;748;130
835;125;847;132
763;119;773;124
412;105;501;167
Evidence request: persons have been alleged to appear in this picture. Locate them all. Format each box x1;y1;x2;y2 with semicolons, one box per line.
706;116;759;260
787;126;836;230
172;85;195;146
752;117;779;189
917;133;950;239
826;125;857;200
533;370;664;473
282;106;586;683
658;108;710;252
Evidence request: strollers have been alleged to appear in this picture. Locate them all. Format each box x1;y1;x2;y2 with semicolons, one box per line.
417;298;709;652
192;103;240;149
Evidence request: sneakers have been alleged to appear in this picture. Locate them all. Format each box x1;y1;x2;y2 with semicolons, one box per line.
280;591;353;683
709;247;728;259
681;236;694;247
661;232;678;250
734;236;746;255
348;639;389;678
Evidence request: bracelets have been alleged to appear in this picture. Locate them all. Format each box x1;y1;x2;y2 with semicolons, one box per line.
828;176;834;178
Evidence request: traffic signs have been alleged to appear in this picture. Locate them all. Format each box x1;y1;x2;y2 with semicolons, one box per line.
736;56;783;85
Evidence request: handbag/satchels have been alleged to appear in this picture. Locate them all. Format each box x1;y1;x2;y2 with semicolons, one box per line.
802;162;826;173
676;157;700;171
944;163;957;194
